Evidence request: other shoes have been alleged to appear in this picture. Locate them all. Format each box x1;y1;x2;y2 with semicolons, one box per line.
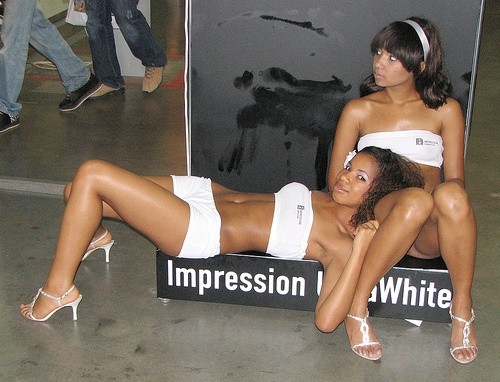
58;72;102;112
0;110;20;133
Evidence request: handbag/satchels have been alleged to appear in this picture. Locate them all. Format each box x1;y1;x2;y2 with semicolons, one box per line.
65;0;120;29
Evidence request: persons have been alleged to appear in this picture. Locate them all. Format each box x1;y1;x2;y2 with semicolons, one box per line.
328;17;479;363
19;146;425;334
0;0;102;134
85;0;167;99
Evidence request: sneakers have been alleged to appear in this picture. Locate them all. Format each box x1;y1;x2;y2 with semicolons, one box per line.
141;65;163;94
89;83;125;100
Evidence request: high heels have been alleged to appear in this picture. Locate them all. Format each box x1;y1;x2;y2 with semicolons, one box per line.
449;301;478;364
343;307;383;361
19;284;82;322
80;228;114;263
